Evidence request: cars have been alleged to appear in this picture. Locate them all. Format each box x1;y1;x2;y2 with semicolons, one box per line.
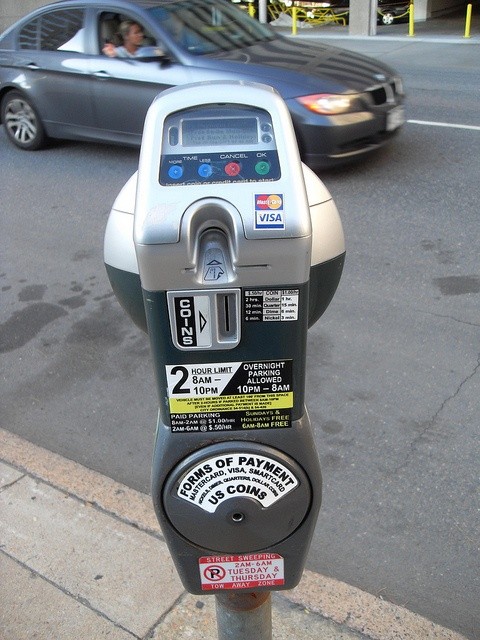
0;0;407;169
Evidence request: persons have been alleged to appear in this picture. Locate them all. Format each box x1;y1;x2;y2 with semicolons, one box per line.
102;20;163;58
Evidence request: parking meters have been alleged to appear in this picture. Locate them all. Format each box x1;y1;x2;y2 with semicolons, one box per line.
102;80;346;634
